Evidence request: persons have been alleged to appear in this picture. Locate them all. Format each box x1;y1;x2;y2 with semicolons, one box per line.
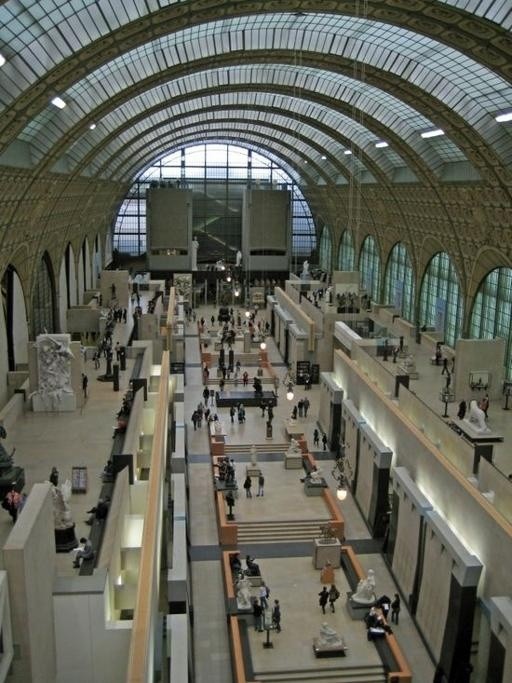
73;537;92;567
305;374;312;391
435;349;450;375
482;393;489;416
103;460;114;474
393;347;399;363
81;373;88;397
50;465;59;486
365;590;400;632
318;585;340;614
191;386;327;450
93;324;121;368
252;582;282;633
307;285;368;311
191;308;269;391
107;307;127;323
84;496;111;526
212;457;265;498
4;489;22;524
